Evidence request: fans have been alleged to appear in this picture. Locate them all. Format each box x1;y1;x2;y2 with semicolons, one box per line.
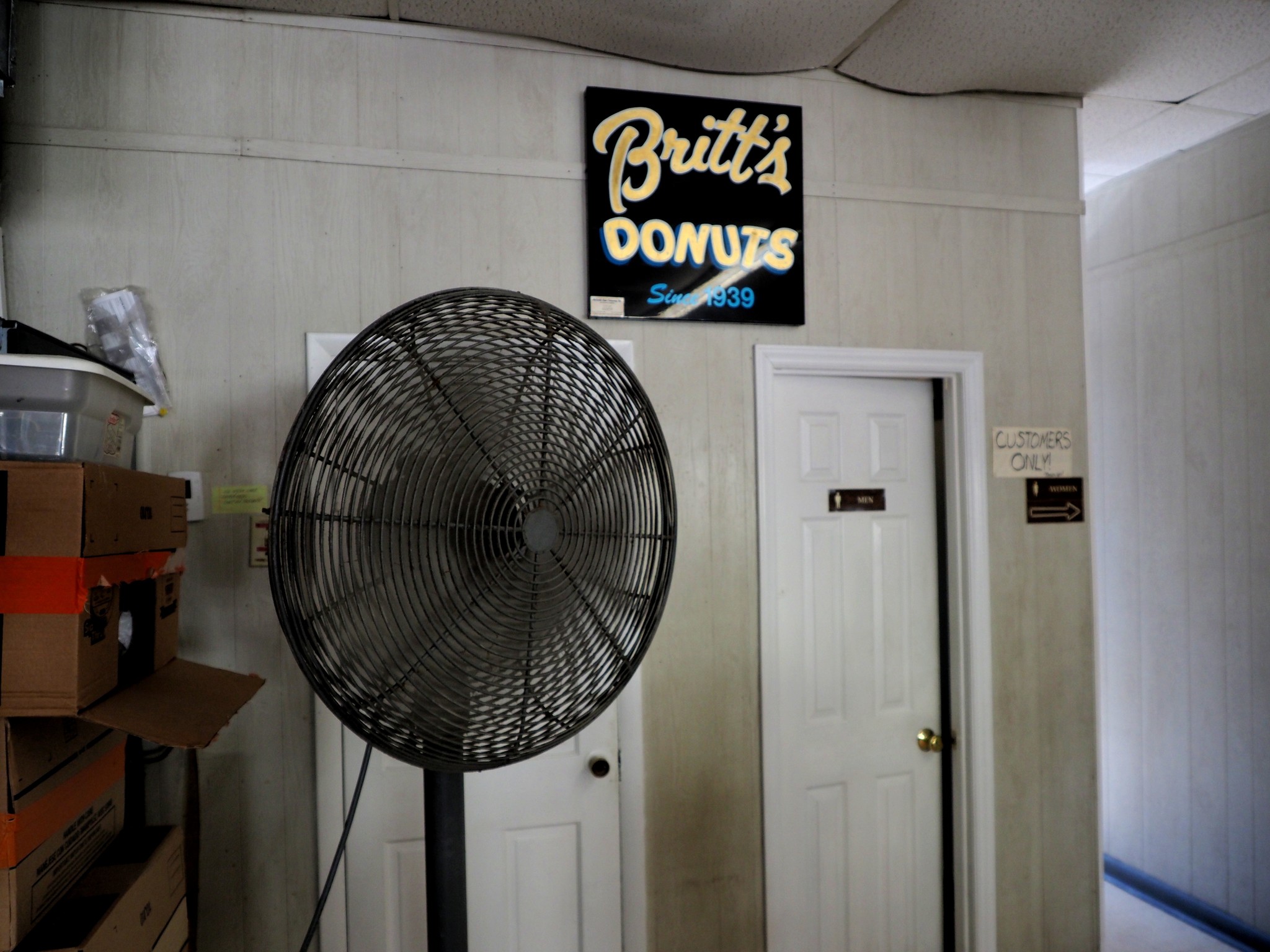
270;288;679;952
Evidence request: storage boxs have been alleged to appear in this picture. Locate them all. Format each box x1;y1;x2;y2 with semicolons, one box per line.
0;352;266;952
